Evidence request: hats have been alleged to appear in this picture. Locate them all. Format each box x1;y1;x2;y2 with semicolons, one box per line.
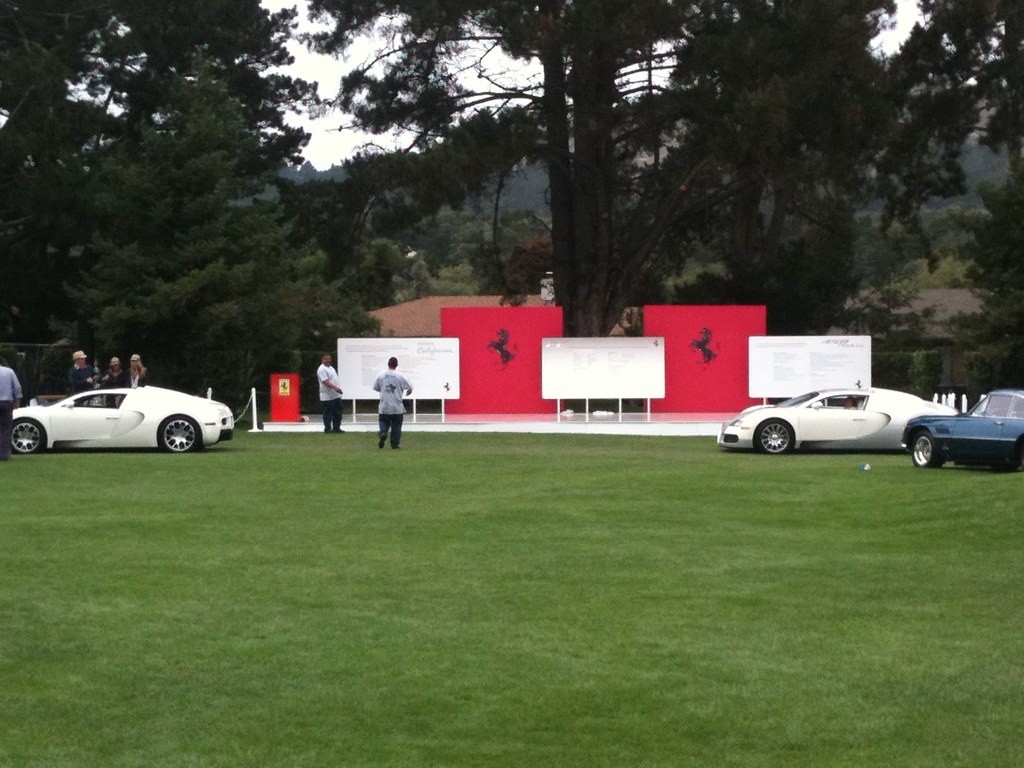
109;357;120;367
131;354;140;362
72;350;87;360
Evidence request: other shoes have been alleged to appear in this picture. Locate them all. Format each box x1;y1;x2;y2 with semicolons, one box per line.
392;446;400;449
325;428;345;433
379;434;386;449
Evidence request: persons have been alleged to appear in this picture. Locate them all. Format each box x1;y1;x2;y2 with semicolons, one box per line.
843;397;857;409
0;356;21;461
317;354;346;433
372;357;413;449
100;356;131;389
69;351;102;397
129;354;146;388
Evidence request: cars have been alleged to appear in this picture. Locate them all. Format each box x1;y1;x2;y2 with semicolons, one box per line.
717;387;957;455
899;388;1024;471
10;385;234;454
